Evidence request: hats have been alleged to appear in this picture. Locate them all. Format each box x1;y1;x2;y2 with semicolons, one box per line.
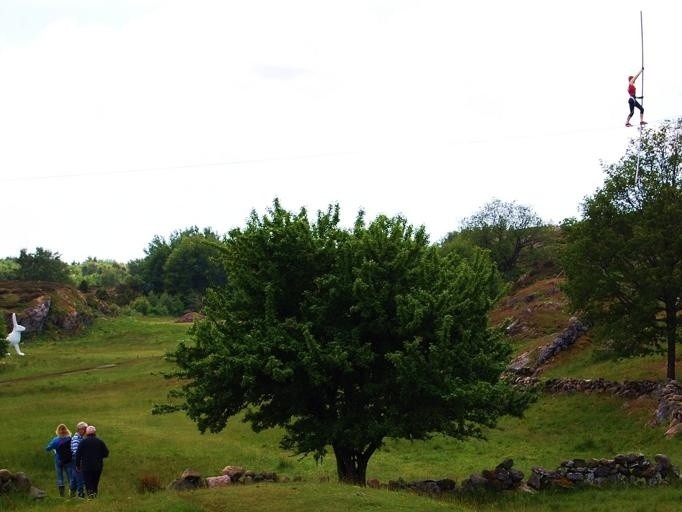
86;426;96;435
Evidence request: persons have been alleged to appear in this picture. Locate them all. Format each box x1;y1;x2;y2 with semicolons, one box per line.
68;421;88;498
75;425;109;499
624;67;648;128
46;423;73;496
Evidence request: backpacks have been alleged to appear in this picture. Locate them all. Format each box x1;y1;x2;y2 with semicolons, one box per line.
59;437;72;463
